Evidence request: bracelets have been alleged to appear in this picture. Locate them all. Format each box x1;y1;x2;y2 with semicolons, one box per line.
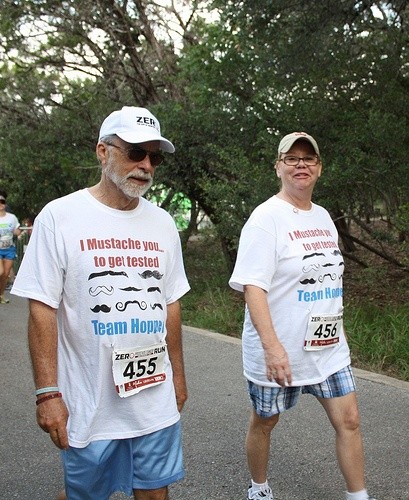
35;387;62;405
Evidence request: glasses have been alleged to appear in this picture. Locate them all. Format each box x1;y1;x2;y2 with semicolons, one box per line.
276;155;320;167
0;200;6;204
106;142;165;167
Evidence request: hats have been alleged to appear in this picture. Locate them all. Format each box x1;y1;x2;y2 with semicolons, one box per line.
98;104;175;154
278;132;320;157
0;195;5;200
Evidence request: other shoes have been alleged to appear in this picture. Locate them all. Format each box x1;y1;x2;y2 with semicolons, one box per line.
246;484;274;500
0;295;10;304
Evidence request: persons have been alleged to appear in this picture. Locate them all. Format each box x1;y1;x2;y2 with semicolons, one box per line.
9;105;192;500
228;132;376;500
0;196;20;304
